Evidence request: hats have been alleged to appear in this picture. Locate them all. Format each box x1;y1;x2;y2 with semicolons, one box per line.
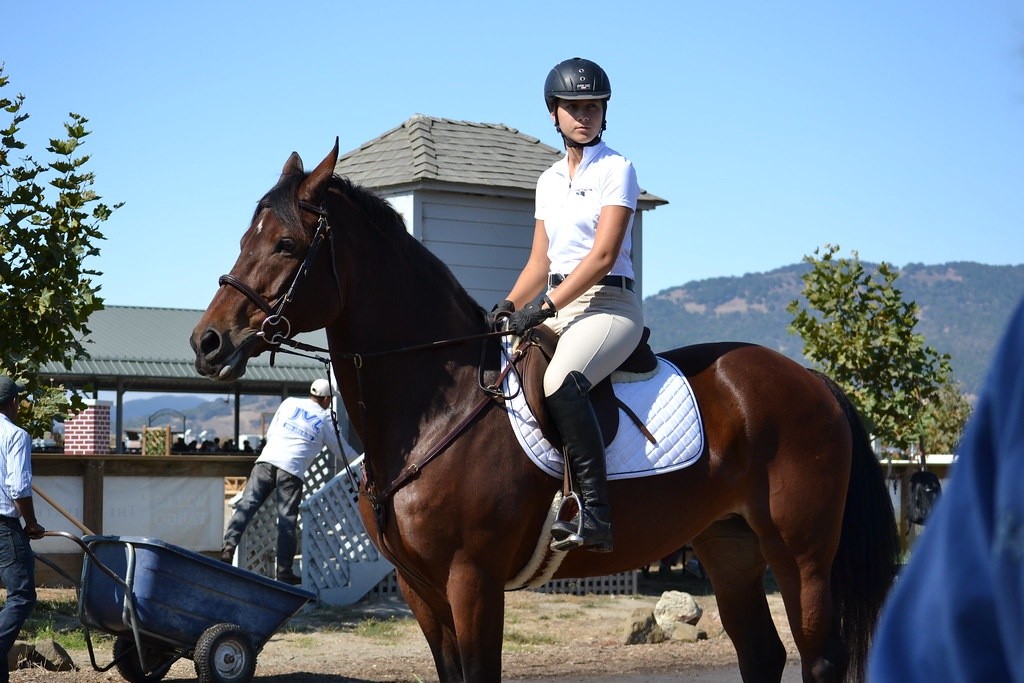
0;376;26;403
310;379;341;398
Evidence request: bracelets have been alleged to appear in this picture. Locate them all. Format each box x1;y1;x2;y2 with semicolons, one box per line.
545;296;557;313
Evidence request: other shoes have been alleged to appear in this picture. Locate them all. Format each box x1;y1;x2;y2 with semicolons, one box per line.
277;575;301;585
220;553;233;565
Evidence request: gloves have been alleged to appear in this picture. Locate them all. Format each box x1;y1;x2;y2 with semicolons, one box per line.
490;299;515;321
508;293;558;339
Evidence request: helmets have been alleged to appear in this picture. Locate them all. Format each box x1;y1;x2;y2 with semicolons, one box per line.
544;57;612;149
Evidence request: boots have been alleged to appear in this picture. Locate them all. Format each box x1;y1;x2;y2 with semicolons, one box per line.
544;370;613;553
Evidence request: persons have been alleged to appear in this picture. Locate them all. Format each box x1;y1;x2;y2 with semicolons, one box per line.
490;57;643;553
0;376;45;683
221;379;360;585
174;437;266;455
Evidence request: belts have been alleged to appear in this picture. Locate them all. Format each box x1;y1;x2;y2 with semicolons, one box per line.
546;272;636;294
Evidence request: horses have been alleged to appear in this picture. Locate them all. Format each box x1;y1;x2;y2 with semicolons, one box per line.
187;136;904;683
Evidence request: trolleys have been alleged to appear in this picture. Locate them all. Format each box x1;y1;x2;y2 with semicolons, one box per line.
27;532;315;683
